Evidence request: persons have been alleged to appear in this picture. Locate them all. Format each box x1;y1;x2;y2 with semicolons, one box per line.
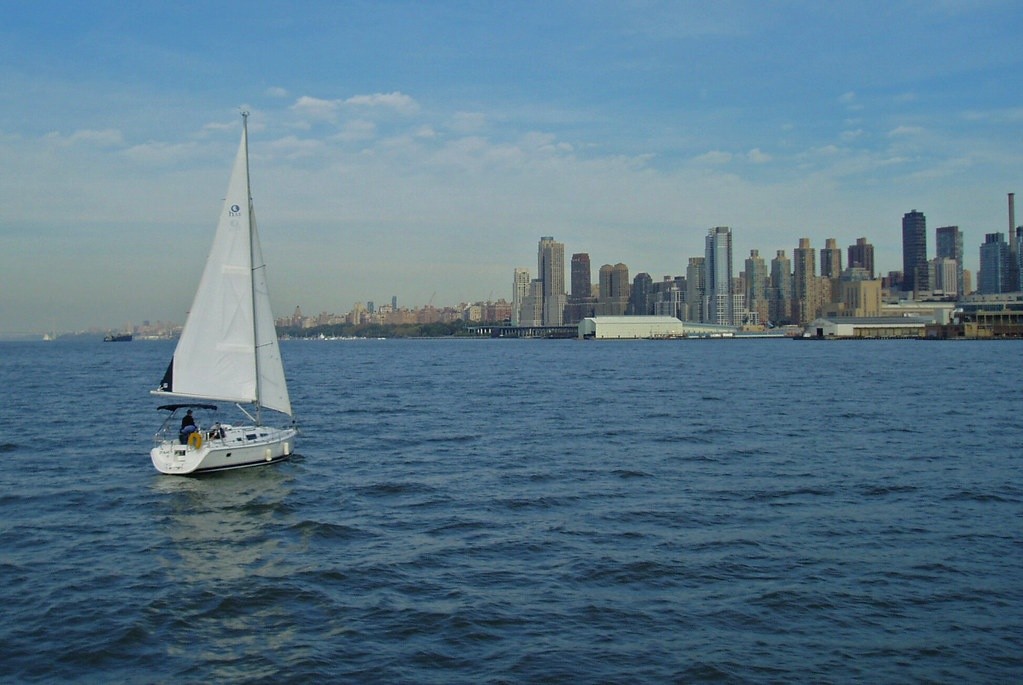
206;422;224;440
181;410;198;455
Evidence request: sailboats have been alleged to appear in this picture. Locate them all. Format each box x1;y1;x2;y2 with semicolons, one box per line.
150;105;301;476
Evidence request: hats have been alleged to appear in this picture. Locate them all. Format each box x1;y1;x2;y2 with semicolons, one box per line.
187;410;192;412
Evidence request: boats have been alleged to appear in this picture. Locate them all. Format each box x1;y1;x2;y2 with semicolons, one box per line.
103;334;134;343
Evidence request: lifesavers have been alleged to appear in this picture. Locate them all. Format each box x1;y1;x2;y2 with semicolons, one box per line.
189;434;201;448
